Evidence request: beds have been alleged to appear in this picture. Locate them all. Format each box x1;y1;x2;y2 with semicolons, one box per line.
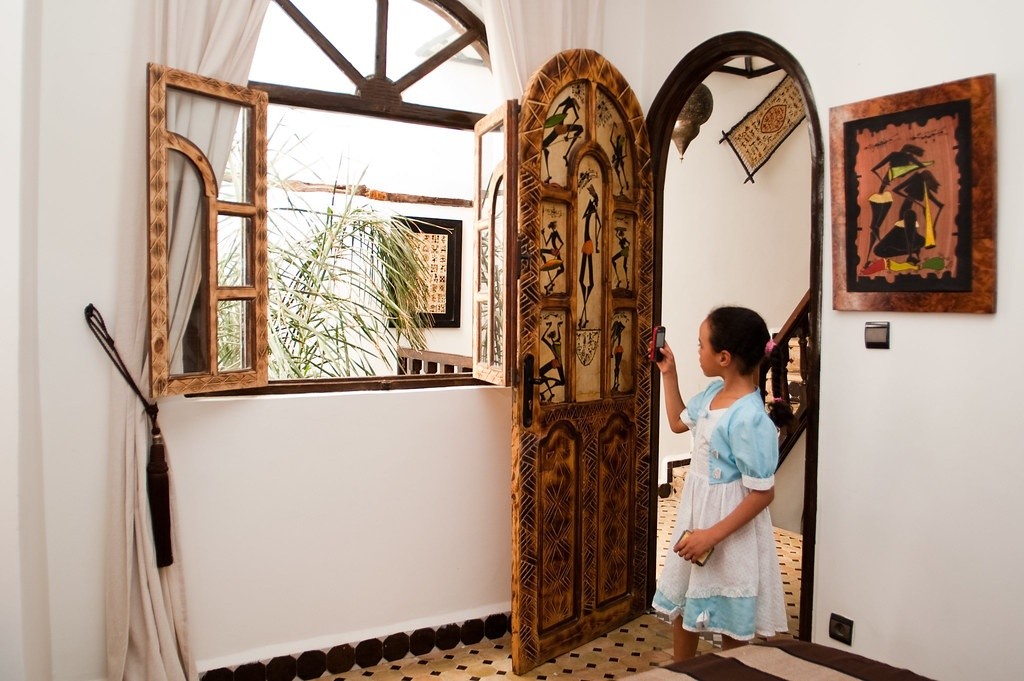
613;628;939;681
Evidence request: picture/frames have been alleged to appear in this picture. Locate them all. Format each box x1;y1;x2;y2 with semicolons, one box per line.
389;217;461;329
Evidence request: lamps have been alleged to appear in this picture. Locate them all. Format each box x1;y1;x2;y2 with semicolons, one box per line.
668;82;714;160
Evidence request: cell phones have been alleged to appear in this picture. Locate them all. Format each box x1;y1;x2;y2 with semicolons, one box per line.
650;325;666;362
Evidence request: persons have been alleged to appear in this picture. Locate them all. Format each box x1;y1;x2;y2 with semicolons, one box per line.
648;306;794;663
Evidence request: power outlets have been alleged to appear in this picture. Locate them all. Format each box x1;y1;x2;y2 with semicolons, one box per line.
829;612;854;646
